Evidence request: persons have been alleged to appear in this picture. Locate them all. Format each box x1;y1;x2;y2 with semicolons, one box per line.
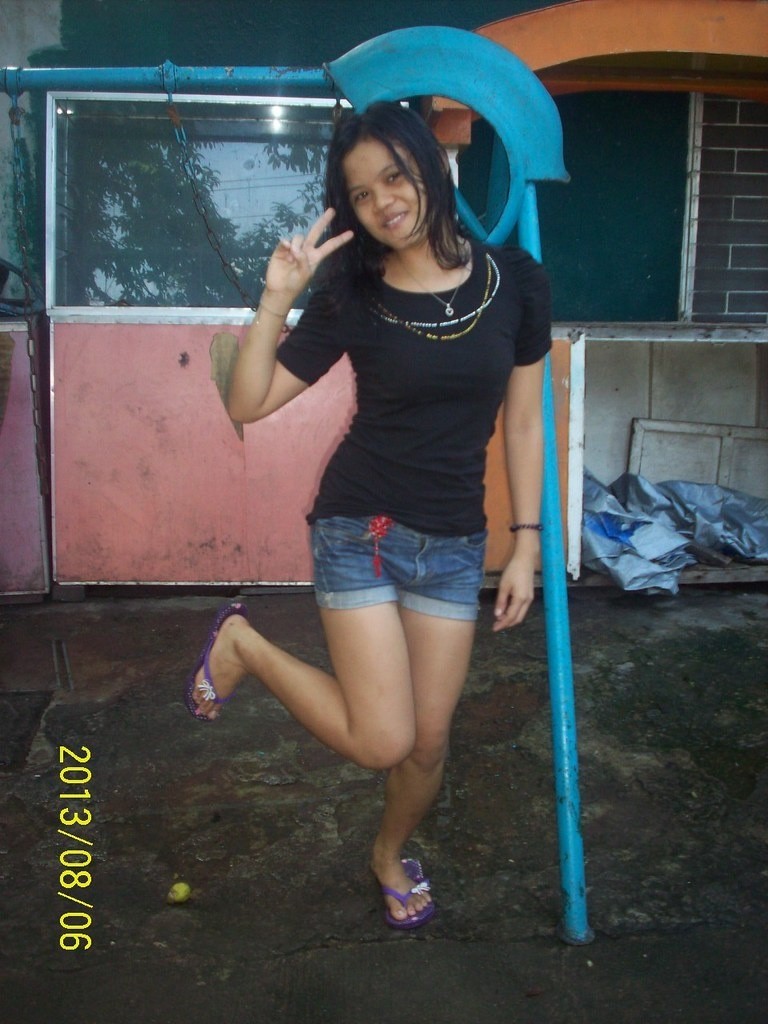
184;102;551;931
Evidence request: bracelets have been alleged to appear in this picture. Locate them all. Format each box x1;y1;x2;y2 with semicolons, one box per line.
509;522;546;535
255;301;288;327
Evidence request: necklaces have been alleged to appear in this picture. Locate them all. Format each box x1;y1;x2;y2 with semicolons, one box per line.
365;240;501;341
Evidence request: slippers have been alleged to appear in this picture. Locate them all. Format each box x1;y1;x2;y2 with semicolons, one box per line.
381;858;435;930
183;602;250;722
